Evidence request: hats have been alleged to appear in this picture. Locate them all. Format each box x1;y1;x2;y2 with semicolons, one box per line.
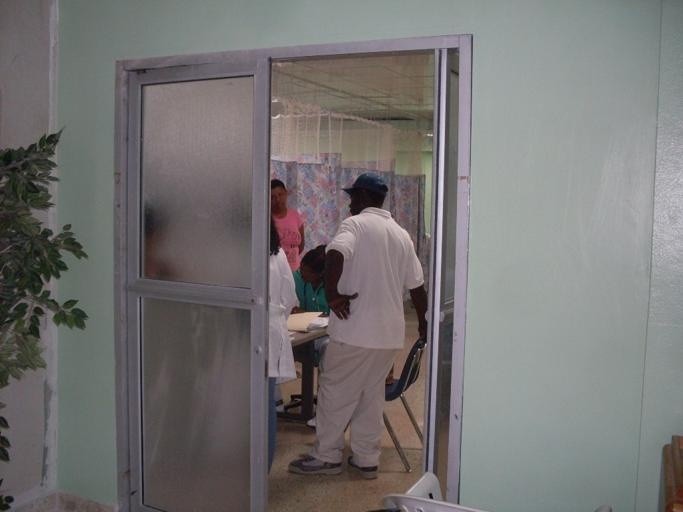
339;172;389;198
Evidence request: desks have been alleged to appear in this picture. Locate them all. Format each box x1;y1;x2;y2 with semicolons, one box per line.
280;311;331;426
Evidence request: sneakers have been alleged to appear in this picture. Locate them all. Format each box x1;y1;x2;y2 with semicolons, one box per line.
288;454;343;476
346;454;378;480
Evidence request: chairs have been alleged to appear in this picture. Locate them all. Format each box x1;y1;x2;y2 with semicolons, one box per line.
342;334;427;472
380;472;613;512
284;346;322;412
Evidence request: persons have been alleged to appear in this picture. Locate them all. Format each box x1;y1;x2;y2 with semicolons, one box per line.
267;212;300;474
270;179;306;271
274;243;327;428
286;172;428;479
